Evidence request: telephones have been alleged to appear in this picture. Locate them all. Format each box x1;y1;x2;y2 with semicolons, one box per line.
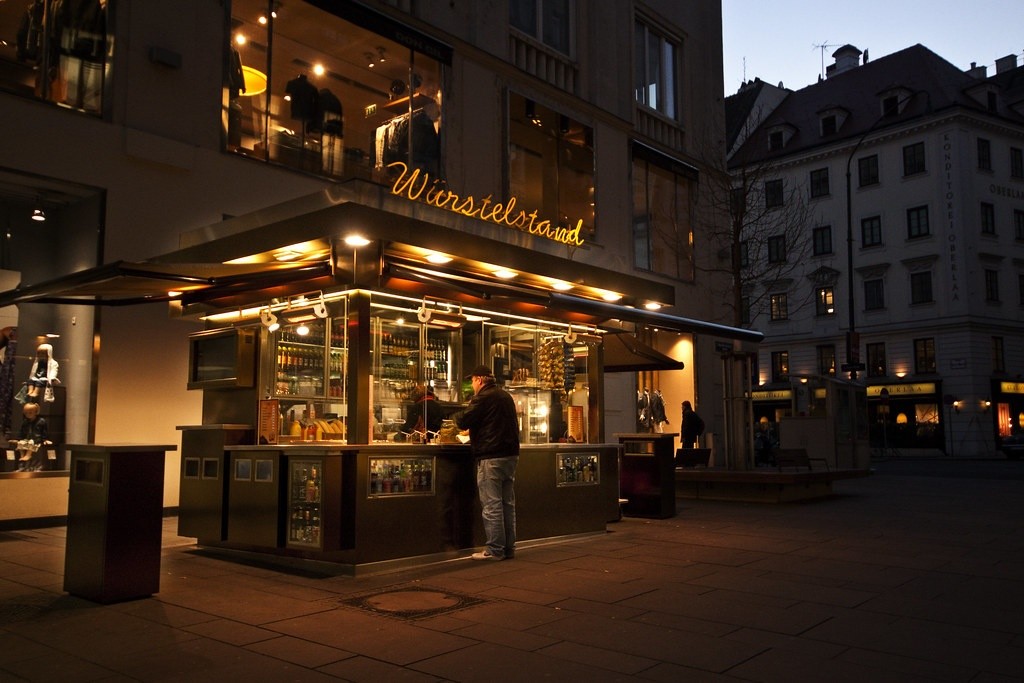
492;356;513;380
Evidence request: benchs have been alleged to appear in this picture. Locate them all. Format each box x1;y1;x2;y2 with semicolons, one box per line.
773;448;830;472
674;449;712;468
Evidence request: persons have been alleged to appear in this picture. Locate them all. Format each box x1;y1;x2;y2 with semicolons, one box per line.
453;365;520;561
637;388;670;431
284;73;345;139
9;402;52;461
679;401;697;449
399;386;443;443
27;344;62;401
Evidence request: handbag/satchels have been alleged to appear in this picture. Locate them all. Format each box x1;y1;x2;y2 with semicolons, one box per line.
404;416;425;441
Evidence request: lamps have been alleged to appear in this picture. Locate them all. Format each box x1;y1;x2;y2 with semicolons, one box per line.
365;53;374;67
281;290;328;323
418;295;467;327
564;321;602;346
377;47;386;62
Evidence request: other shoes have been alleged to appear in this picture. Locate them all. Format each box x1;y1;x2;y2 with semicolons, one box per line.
506;551;514;558
472;550;505;562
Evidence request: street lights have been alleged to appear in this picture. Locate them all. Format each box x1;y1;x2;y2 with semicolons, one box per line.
841;87;935;389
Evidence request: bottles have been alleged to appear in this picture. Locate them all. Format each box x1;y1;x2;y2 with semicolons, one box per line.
290;469;320;543
381;333;419;400
559;457;597;483
370;460;432;493
424;336;447;380
277;324;348;397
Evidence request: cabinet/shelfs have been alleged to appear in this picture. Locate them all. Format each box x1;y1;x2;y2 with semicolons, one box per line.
273;318;453;399
223;444;624;563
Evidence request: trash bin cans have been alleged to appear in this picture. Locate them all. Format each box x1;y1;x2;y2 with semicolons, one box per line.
613;432;681;521
45;442;178;603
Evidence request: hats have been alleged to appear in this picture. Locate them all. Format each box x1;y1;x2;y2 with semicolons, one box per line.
759;417;768;424
464;365;494;379
682;401;691;408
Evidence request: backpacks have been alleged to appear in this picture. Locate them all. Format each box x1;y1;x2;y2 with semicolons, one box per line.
684;409;705;437
